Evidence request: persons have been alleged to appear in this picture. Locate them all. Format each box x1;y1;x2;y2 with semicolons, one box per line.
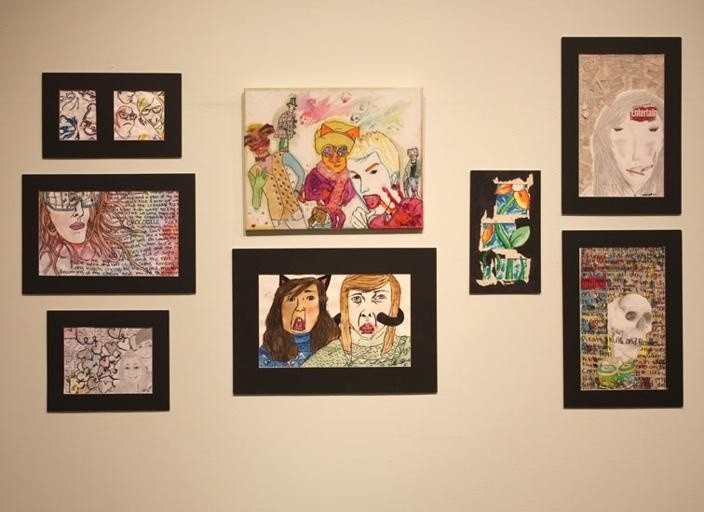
38;191;151;276
104;328;155;393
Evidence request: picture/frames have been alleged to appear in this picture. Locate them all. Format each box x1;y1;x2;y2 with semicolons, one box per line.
232;247;439;396
22;174;196;295
42;72;182;159
561;229;684;409
47;311;170;413
561;36;681;215
